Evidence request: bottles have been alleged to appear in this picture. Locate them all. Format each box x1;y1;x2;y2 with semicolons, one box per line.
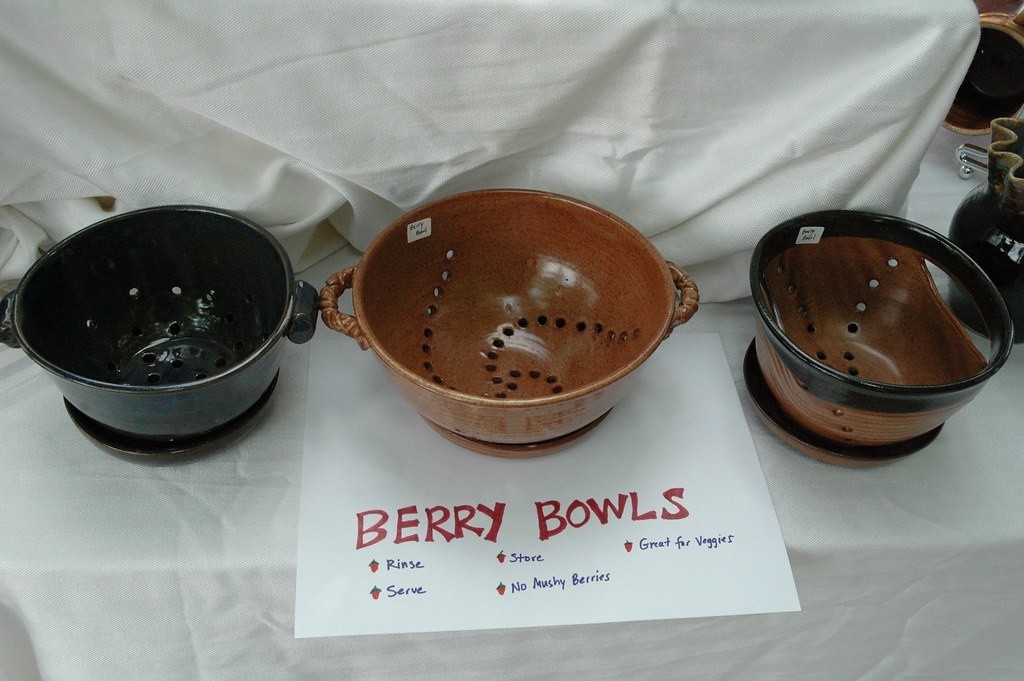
947;118;1024;344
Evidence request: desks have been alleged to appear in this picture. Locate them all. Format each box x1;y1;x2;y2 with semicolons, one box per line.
0;119;1024;681
